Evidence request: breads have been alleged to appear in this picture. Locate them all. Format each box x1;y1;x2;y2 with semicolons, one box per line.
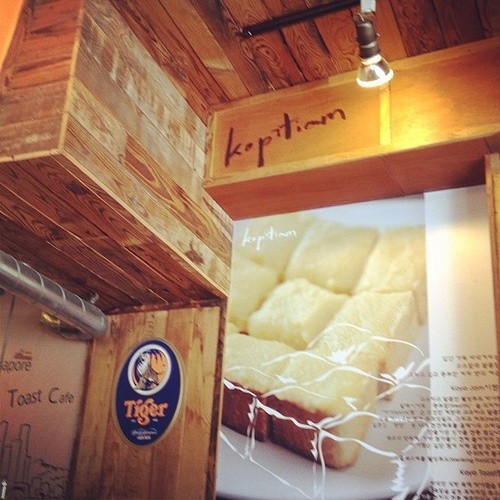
220;213;426;471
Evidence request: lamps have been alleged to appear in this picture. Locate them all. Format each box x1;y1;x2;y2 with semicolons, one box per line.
352;12;393;91
